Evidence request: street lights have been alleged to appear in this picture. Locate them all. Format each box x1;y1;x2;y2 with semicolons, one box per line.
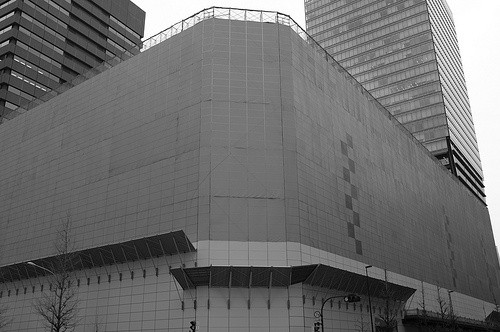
365;265;374;332
28;261;57;332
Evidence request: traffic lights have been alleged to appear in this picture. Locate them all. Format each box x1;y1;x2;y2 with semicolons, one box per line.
343;295;361;302
